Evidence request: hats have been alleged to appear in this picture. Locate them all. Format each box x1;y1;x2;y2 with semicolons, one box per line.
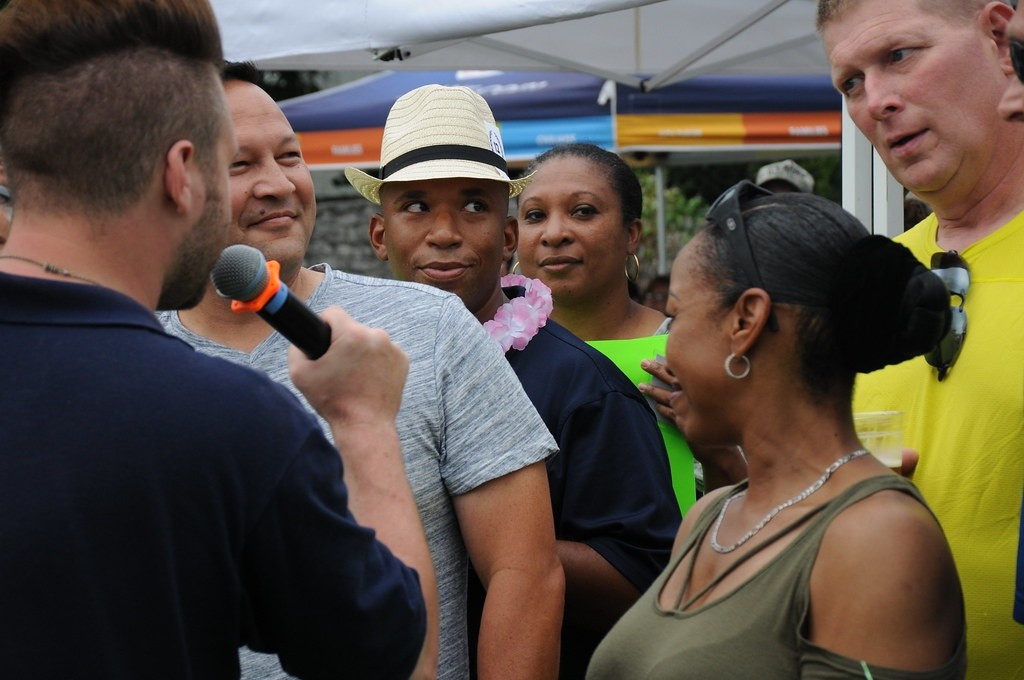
345;84;538;205
756;160;814;193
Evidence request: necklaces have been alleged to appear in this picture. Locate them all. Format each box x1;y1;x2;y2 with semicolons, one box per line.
713;449;870;553
484;274;556;347
0;255;101;288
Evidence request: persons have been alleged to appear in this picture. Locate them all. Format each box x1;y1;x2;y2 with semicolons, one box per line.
817;0;1024;680
0;0;439;680
585;189;967;680
141;64;700;680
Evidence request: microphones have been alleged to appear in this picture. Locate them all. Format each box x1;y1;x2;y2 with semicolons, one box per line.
210;243;332;360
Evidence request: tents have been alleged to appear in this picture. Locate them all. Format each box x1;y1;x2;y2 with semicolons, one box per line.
213;2;901;239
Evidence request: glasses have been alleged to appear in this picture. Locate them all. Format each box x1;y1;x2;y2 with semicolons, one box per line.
1008;39;1024;83
0;185;12;204
705;181;779;333
643;292;667;302
924;250;970;382
